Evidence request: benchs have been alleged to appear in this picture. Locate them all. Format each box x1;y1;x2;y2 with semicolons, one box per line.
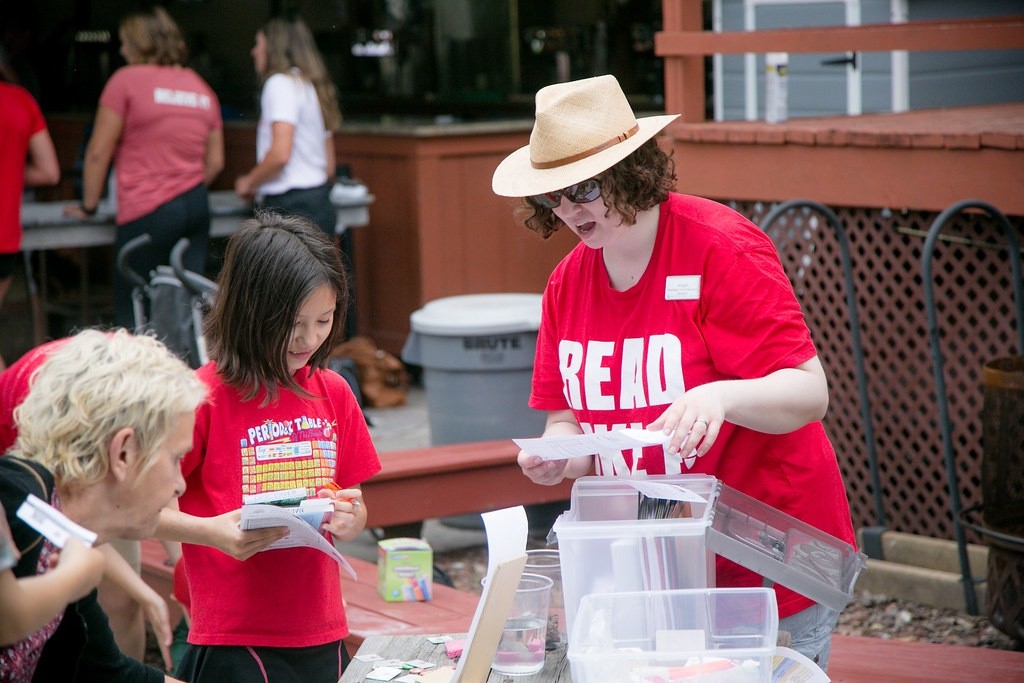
363;439;573;529
141;537;484;657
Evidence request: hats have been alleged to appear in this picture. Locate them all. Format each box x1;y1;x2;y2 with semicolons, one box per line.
492;75;682;198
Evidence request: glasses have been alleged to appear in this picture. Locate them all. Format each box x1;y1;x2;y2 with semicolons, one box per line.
529;171;614;208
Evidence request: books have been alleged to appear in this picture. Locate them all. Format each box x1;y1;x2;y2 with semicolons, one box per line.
244;487;335;538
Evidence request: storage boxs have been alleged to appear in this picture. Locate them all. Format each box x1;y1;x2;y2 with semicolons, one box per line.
377;537;434;605
552;474;869;643
565;587;779;683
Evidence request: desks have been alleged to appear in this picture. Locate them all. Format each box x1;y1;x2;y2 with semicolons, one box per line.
20;184;379;345
337;632;572;683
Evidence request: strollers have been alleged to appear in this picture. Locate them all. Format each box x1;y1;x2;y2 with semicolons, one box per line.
113;233;364;411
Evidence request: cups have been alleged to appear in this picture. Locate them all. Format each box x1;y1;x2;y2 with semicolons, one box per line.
481;572;554;677
522;549;568;650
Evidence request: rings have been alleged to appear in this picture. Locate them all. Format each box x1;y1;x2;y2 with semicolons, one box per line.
695;419;708;425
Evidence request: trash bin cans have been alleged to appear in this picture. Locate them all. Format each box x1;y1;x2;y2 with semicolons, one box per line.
401;293;572;532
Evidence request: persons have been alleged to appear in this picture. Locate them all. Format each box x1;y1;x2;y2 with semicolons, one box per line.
0;324;216;683
491;73;860;673
150;206;383;683
97;496;192;673
0;501;105;647
63;6;224;370
0;82;61;368
235;15;342;242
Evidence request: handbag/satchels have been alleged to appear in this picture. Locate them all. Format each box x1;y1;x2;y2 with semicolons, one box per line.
327;359;373;425
330;337;407;406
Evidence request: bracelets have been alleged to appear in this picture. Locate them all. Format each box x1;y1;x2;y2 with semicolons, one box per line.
79;201;97;216
